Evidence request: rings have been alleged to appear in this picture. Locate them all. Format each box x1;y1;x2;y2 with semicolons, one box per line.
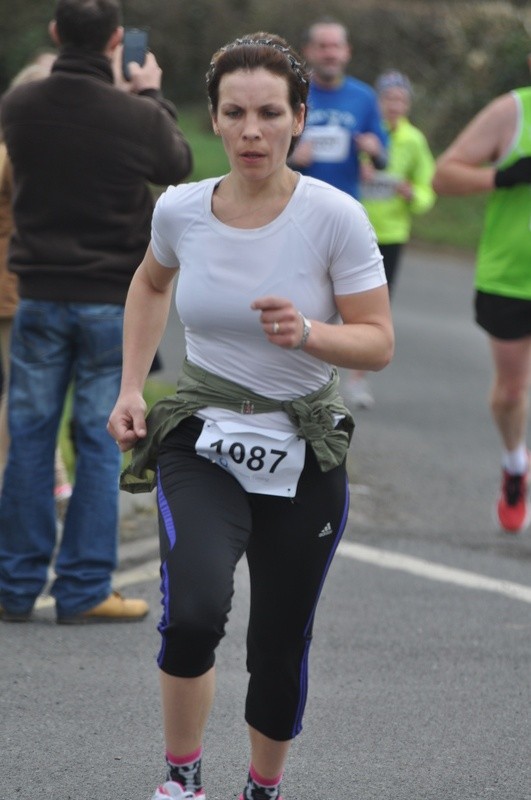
273;322;278;333
116;440;119;445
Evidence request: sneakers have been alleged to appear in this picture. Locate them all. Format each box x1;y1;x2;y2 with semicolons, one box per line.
498;455;527;533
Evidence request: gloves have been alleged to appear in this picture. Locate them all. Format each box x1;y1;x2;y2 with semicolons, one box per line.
494;157;531;187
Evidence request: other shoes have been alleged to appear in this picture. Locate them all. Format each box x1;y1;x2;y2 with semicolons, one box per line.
0;605;30;622
52;481;74;502
348;383;373;406
151;781;206;800
58;592;148;623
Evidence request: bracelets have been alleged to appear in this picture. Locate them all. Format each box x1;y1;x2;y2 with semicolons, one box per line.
291;311;311;348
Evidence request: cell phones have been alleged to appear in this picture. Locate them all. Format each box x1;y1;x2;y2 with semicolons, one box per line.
121;26;152;82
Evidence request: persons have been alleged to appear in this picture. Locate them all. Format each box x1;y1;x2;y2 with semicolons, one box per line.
358;69;435;297
285;21;389;410
429;86;531;533
0;0;192;625
104;32;393;800
